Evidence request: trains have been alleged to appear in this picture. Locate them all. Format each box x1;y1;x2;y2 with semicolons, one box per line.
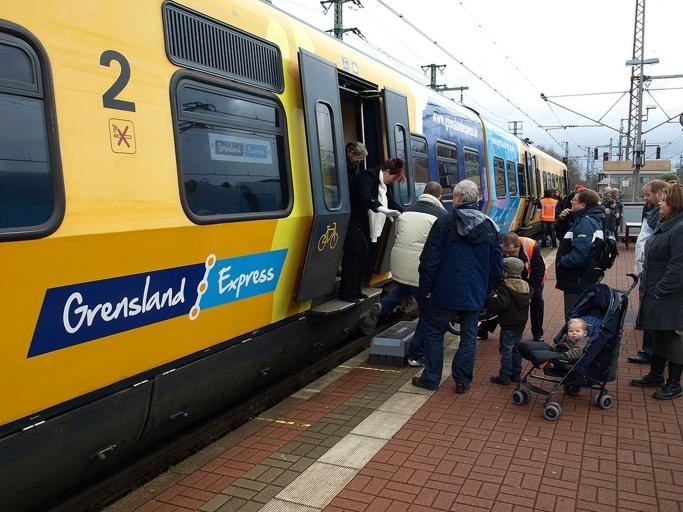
0;0;573;512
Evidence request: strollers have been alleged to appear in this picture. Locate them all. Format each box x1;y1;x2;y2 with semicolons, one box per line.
511;267;639;423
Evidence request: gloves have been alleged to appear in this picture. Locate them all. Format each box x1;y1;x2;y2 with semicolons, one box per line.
376;205;402;223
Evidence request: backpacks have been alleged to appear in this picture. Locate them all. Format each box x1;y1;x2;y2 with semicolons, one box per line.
579;215;618;272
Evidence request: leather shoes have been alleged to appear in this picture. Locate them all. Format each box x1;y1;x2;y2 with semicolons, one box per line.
477;325;488;340
411;376;440;391
455;382;471;395
651;380;683;400
627;350;654;365
630;372;666;388
338;293;369;302
532;335;544;341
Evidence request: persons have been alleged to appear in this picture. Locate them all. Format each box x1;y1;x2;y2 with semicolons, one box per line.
365;180;546;393
518;318;590;369
627;174;683;400
555;188;606;315
335;142;404;303
537;184;624;248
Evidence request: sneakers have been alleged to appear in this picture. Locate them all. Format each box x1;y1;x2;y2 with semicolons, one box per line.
490;372;523;386
407;354;431;368
361;302;382;336
